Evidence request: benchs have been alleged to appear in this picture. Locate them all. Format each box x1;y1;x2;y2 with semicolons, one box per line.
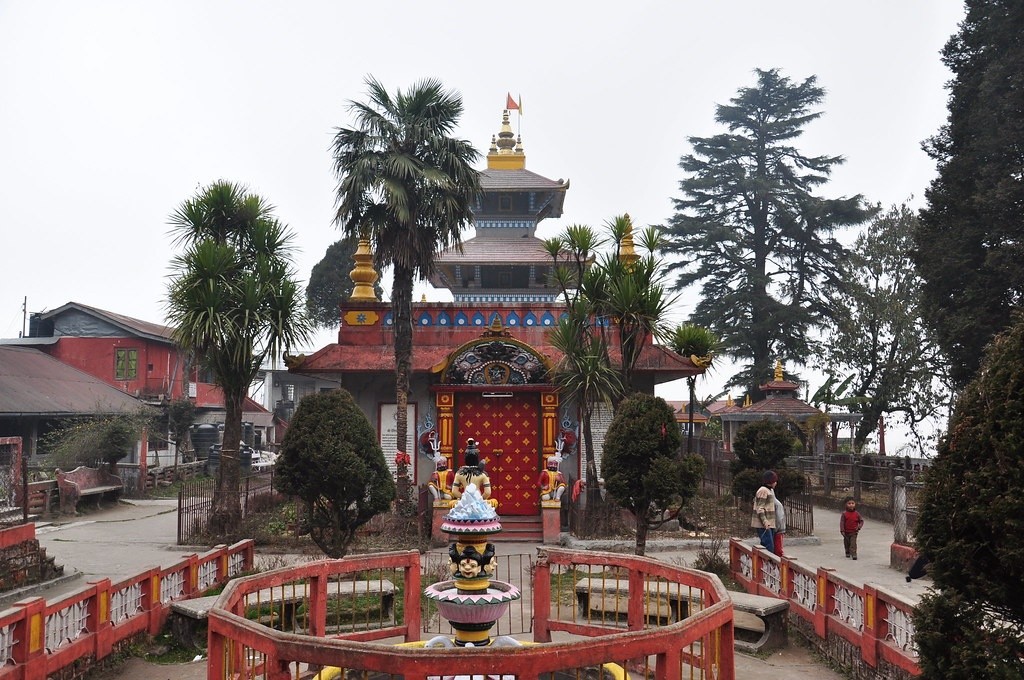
572;577;790;655
170;580;400;654
54;465;125;515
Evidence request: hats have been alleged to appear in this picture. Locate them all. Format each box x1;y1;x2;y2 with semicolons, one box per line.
763;470;778;484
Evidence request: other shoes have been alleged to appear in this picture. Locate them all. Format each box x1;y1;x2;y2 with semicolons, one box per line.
846;552;850;557
852;555;857;560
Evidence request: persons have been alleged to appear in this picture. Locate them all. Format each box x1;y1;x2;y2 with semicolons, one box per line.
446;542;497;579
428;456;455;500
839;496;864;560
537;455;566;501
448;437;498;510
750;471;786;557
